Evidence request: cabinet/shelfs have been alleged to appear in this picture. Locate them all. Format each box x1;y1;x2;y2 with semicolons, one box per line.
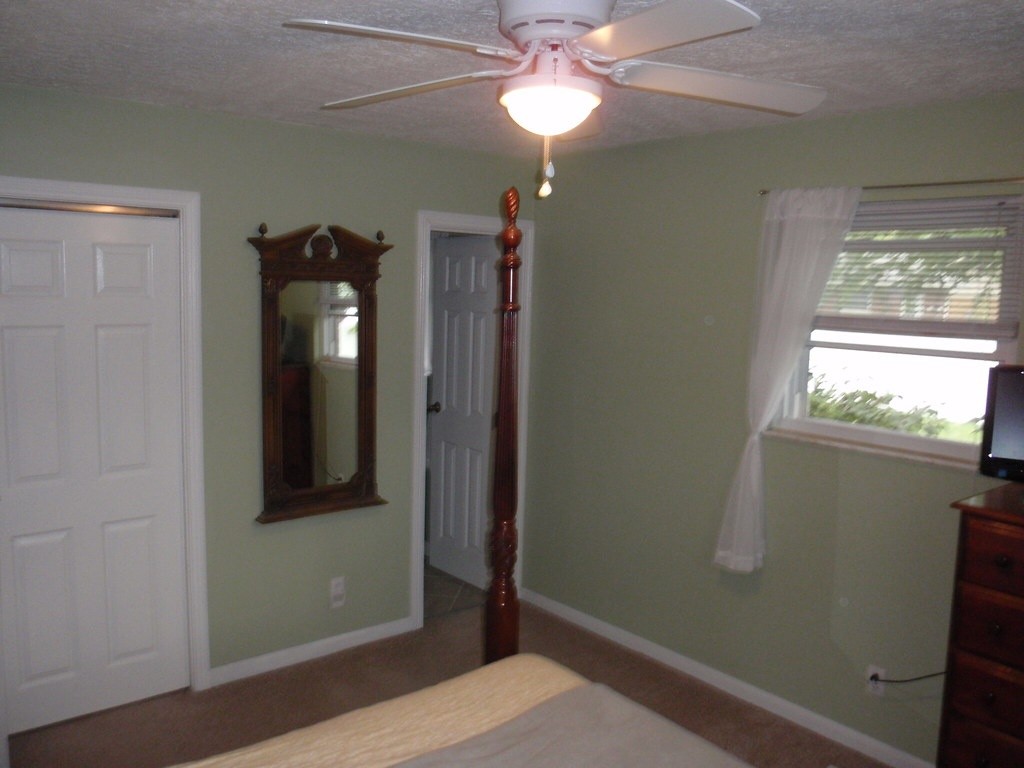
934;483;1024;767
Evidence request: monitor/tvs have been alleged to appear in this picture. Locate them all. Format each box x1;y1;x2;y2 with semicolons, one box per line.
979;363;1024;483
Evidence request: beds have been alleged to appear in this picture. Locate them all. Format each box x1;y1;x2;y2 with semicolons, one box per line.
158;649;754;768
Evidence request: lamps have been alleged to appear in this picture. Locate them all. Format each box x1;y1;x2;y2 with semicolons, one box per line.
498;52;604;198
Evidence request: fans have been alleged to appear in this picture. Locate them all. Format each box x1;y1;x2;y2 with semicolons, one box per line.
283;0;827;116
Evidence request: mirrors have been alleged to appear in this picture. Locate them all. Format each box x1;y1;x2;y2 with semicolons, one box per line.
247;222;394;524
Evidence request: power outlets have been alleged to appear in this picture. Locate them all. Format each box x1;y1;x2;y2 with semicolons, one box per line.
866;664;886;696
